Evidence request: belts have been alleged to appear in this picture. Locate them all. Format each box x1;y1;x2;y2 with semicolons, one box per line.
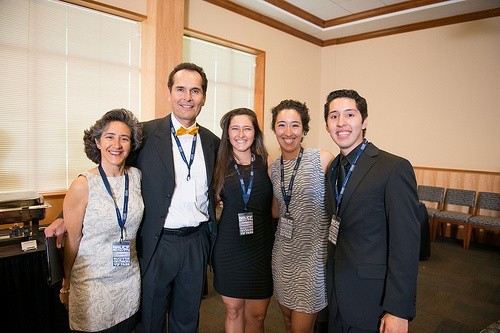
162;222;208;237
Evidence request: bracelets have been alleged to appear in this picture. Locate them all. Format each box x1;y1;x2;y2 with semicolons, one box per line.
57;288;71;292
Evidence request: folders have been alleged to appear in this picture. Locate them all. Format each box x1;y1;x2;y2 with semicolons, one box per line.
45;236;64;286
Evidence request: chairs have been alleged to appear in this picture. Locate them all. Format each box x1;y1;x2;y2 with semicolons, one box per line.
466;192;500;251
433;188;476;248
418;185;444;240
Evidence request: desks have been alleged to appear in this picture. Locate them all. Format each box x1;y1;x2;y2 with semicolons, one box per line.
0;224;51;262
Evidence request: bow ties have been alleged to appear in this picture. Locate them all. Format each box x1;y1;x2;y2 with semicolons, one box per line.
175;126;199;135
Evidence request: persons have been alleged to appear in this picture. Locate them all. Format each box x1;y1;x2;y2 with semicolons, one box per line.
44;62;221;333
319;88;421;333
211;107;278;333
58;107;146;333
217;100;337;333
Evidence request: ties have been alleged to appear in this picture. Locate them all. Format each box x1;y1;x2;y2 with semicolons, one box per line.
337;154;349;196
